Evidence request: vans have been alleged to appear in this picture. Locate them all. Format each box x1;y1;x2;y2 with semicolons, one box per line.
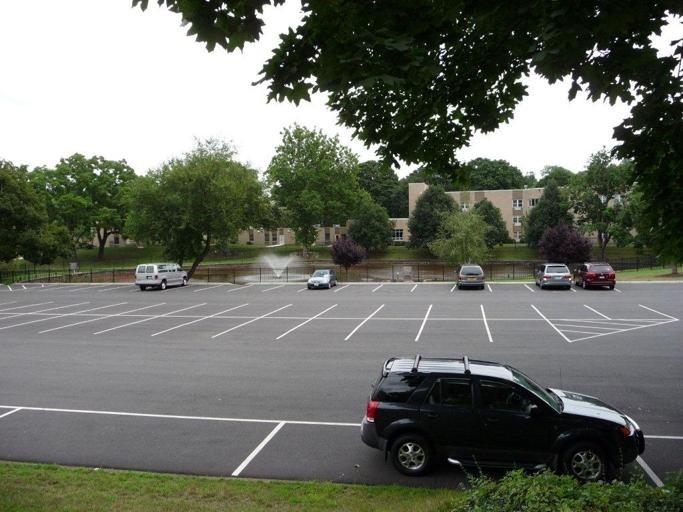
134;262;189;291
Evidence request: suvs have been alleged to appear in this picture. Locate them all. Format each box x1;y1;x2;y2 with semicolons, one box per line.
360;354;645;485
573;262;616;290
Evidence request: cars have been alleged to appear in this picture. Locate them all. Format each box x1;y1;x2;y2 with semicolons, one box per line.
535;263;572;289
456;265;485;289
307;269;337;290
